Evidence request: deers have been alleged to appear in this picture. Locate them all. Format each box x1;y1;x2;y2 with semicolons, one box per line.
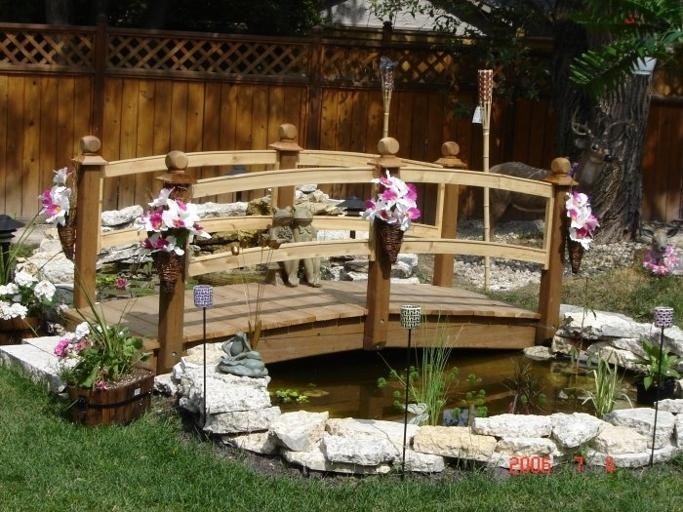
635;220;683;276
481;105;634;262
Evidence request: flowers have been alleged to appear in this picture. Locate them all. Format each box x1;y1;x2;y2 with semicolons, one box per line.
358;168;421;232
132;186;212;259
37;167;72;227
0;270;55;321
54;270;153;388
565;191;601;253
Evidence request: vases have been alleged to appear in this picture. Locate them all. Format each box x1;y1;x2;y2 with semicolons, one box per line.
150;247;183;296
567;233;584;275
0;311;45;346
56;208;74;262
64;363;153;428
375;215;404;263
